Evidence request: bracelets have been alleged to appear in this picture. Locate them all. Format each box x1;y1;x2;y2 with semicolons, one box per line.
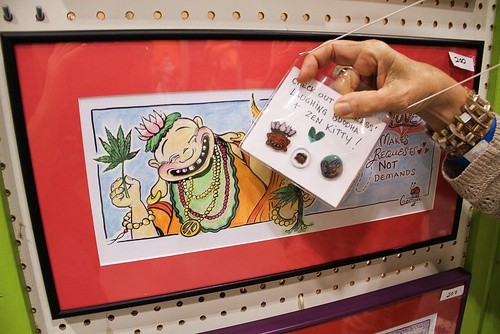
425;83;493;158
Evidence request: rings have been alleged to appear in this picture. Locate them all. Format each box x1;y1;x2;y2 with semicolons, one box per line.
333;63;359;79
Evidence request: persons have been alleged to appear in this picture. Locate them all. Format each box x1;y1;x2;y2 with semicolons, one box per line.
298;38;500;220
